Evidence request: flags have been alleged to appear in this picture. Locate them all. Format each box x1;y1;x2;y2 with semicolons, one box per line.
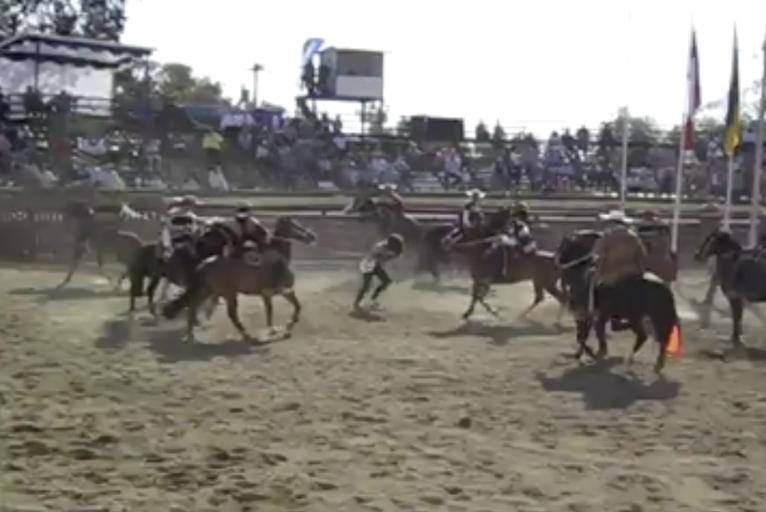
724;31;746;157
682;31;701;152
759;39;766;164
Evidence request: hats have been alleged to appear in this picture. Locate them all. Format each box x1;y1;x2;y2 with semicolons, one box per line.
600;211;633;224
378;183;398;190
466;189;486;199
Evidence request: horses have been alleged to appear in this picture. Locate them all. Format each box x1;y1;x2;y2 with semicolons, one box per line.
57;203;141;289
129;212;318;342
426;198;566;320
694;225;766;342
342;179;422;267
553;227;681;374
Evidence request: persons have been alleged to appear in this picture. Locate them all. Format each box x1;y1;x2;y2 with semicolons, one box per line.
0;90;765;329
